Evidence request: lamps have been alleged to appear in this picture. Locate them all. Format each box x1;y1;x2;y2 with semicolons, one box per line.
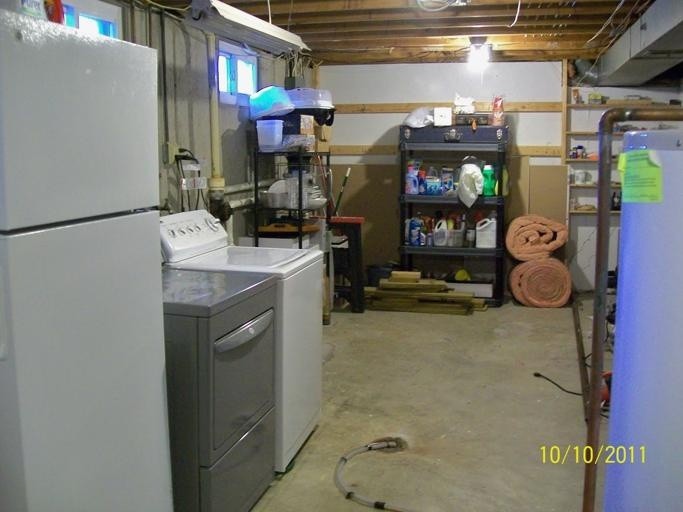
183;0;312;59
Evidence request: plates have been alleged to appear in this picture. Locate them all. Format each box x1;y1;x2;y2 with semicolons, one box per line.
267;180;285;192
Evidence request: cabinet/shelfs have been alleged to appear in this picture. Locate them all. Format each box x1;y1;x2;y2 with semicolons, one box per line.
253;151;333;325
564;102;681;216
399;127;503;310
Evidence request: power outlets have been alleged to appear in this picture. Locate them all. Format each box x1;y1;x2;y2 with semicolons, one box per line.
166;144;186;166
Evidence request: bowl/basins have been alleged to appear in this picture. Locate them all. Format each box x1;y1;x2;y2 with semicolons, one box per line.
258;190;289;209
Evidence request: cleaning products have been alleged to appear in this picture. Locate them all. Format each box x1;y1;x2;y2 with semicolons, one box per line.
481;163;498;196
417;170;429;195
432;215;449;247
408;217;422;246
475;217;498;249
404;166;420;195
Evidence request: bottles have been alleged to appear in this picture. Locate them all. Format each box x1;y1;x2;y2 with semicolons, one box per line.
491;97;504;127
569;145;586;159
460;213;466;231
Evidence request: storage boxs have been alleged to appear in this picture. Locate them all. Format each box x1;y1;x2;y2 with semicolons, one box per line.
248;83;338;155
431;226;463;246
255;234;309;249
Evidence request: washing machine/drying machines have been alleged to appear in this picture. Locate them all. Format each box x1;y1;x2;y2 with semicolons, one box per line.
159;205;323;472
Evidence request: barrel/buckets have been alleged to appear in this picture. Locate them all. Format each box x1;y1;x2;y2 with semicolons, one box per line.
255;117;285;153
